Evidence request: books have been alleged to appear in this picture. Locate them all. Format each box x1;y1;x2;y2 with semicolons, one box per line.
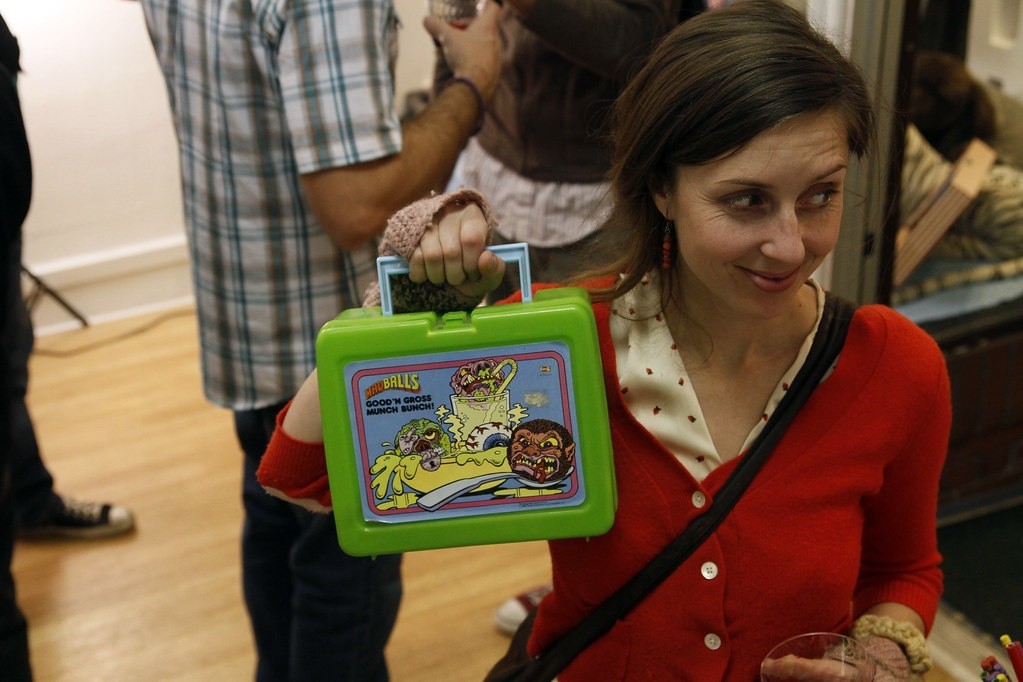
892;137;997;286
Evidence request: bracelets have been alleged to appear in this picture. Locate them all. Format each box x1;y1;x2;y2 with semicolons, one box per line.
851;613;933;682
444;77;485;137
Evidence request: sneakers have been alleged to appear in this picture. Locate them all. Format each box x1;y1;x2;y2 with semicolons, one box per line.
492;581;553;637
15;494;134;541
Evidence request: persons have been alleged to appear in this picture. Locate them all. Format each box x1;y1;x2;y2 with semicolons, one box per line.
0;15;133;682
143;0;515;682
467;0;708;634
257;0;953;682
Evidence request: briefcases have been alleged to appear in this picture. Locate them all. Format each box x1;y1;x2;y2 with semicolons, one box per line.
319;240;619;556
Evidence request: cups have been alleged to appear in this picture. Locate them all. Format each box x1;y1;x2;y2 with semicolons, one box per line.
429;0;477;49
760;633;876;682
